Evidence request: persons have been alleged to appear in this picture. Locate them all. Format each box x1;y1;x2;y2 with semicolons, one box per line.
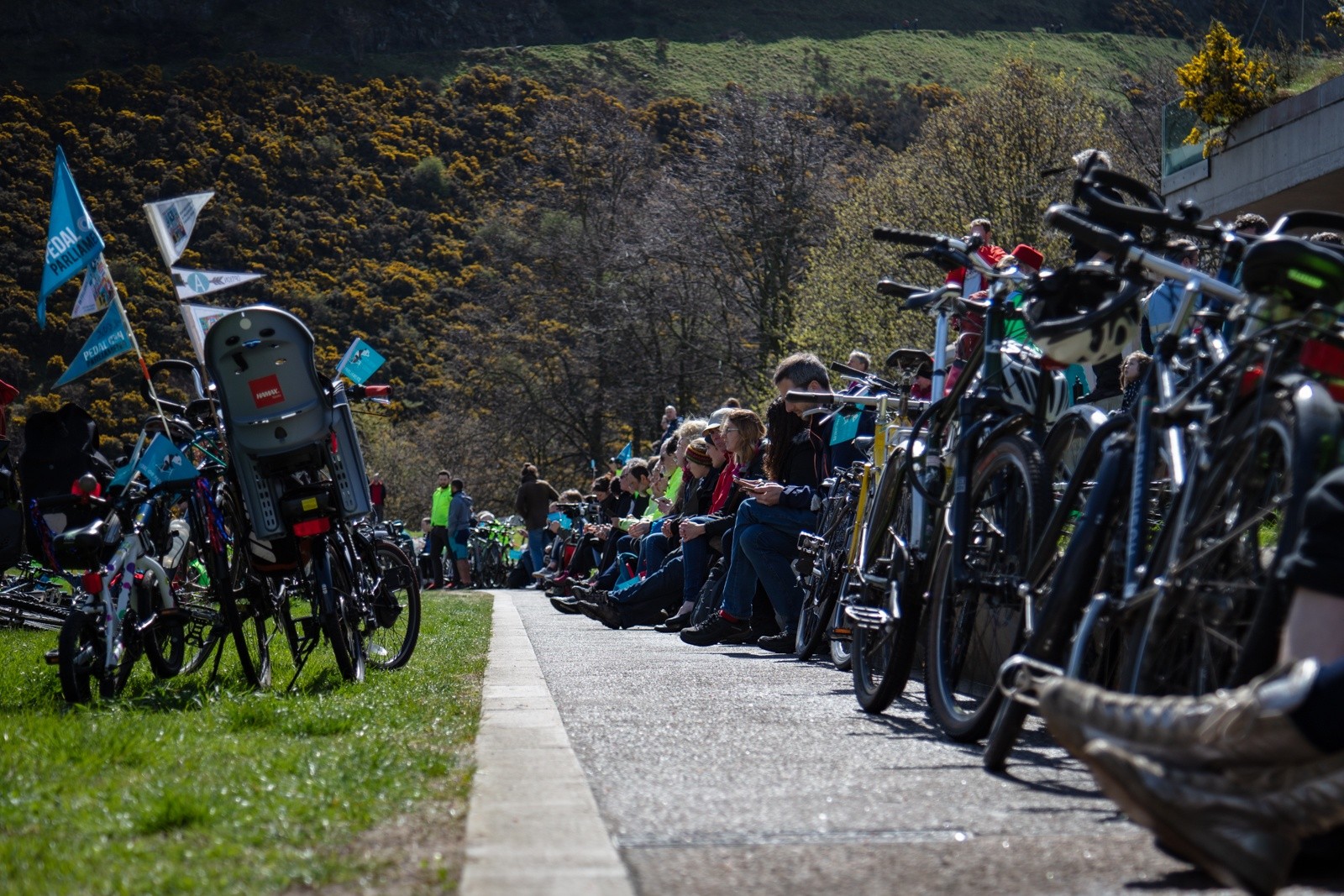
945;219;1008;329
1119;351;1153;413
477;511;528;590
1038;462;1344;896
191;550;212;586
448;478;472;589
427;470;461;590
1068;149;1270;400
394;520;413;558
998;245;1042;346
417;517;434;589
909;362;932;429
514;350;874;653
370;474;386;524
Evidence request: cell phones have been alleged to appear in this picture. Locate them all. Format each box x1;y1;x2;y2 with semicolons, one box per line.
732;475;740;480
742;487;765;494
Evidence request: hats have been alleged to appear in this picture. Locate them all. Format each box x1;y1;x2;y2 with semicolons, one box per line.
684;437;713;466
702;408;732;437
593;474;610;490
522;463;539;478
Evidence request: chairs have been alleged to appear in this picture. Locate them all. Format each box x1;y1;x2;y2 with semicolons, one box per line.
203;304;372;540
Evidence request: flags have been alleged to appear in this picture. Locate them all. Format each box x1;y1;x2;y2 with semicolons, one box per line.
107;433;147;492
138;434;200;505
334;337;387;386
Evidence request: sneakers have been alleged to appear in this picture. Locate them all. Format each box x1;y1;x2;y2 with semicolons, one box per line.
550;595;582;614
449;581;474;590
572;585;614;607
577;600;621;630
680;610;753;647
532;567;571;585
565;576;590;589
757;630;796;653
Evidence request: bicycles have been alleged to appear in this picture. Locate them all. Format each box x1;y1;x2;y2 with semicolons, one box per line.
0;308;516;705
779;160;1344;766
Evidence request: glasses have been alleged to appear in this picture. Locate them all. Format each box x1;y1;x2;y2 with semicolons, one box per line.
722;427;739;435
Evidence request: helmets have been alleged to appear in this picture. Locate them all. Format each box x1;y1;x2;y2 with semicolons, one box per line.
1022;260;1142;365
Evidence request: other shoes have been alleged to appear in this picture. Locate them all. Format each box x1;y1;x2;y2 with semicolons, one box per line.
665;613;690;627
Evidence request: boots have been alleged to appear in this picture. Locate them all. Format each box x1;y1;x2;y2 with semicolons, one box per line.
1086;737;1344;894
1039;656;1319;770
